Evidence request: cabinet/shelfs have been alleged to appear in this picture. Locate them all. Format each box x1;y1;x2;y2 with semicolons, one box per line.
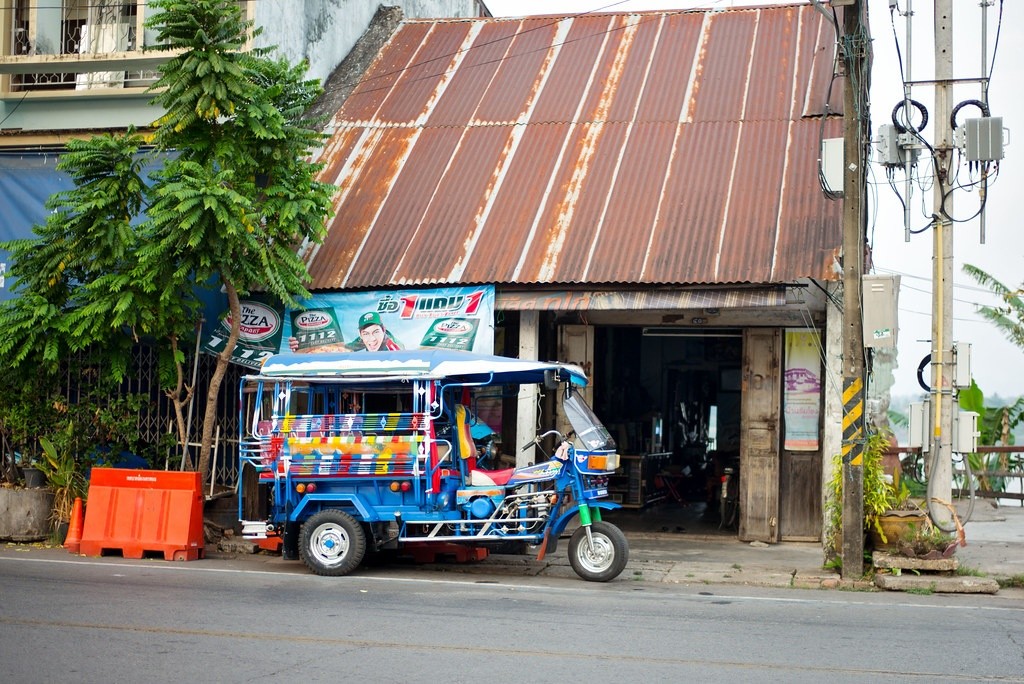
598;451;674;509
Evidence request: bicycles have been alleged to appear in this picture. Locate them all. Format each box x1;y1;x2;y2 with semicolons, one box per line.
717;431;740;535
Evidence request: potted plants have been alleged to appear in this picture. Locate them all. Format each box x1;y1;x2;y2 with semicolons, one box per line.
34;419;89;544
869;466;926;551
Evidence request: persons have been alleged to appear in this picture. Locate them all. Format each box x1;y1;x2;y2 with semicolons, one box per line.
288;312;405;352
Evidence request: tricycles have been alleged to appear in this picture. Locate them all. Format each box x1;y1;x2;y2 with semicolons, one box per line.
236;347;629;582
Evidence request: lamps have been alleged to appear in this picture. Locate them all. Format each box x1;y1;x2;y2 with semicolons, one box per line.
642;327;742;337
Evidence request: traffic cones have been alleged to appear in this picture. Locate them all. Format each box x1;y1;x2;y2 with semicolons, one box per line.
63;497;84;549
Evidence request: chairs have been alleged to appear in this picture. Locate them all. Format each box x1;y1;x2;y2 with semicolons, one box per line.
455;404;516;485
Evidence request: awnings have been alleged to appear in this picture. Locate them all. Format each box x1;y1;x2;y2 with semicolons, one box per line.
429;349;589;389
495;289;786;310
